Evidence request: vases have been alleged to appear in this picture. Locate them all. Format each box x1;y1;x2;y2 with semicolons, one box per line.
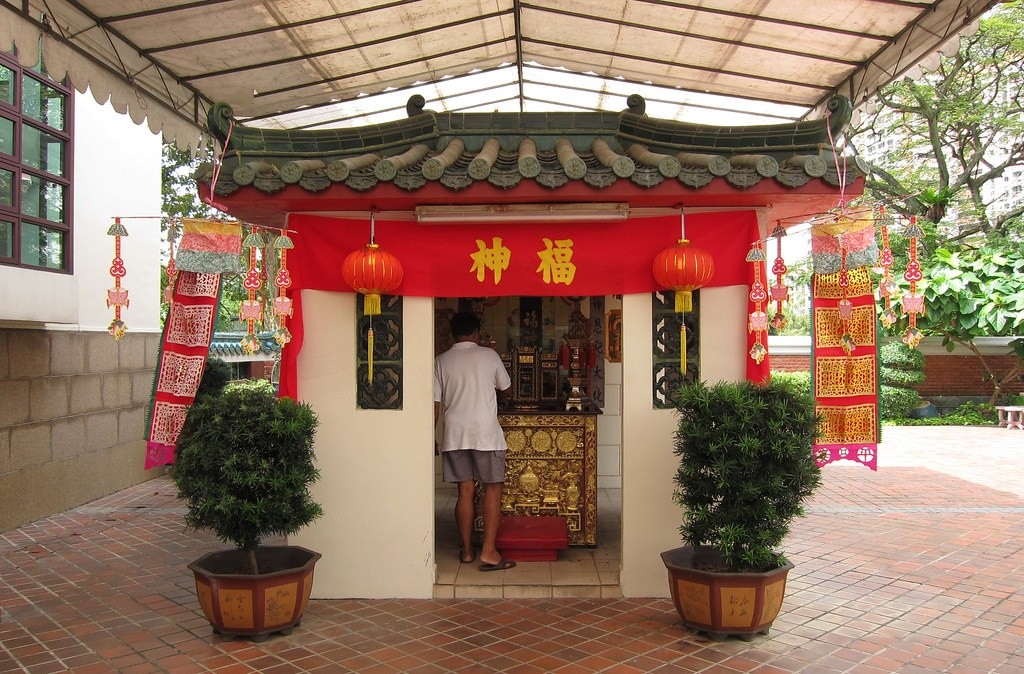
912;400;938;419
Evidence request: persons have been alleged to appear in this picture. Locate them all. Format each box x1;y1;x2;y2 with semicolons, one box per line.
435;313;516;571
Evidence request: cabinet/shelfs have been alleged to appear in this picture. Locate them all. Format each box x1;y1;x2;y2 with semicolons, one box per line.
471;397;604;549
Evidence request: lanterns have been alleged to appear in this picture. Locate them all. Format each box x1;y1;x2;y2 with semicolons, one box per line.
654;239;713;373
343;245;402;383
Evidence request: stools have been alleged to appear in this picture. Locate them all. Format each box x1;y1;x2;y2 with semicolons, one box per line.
995;405;1024;430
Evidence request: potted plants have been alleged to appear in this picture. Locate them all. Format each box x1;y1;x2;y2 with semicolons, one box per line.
169;389;326;644
661;379;825;644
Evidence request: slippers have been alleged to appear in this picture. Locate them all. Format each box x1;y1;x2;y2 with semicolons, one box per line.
459;548;477;563
479;559;516;571
